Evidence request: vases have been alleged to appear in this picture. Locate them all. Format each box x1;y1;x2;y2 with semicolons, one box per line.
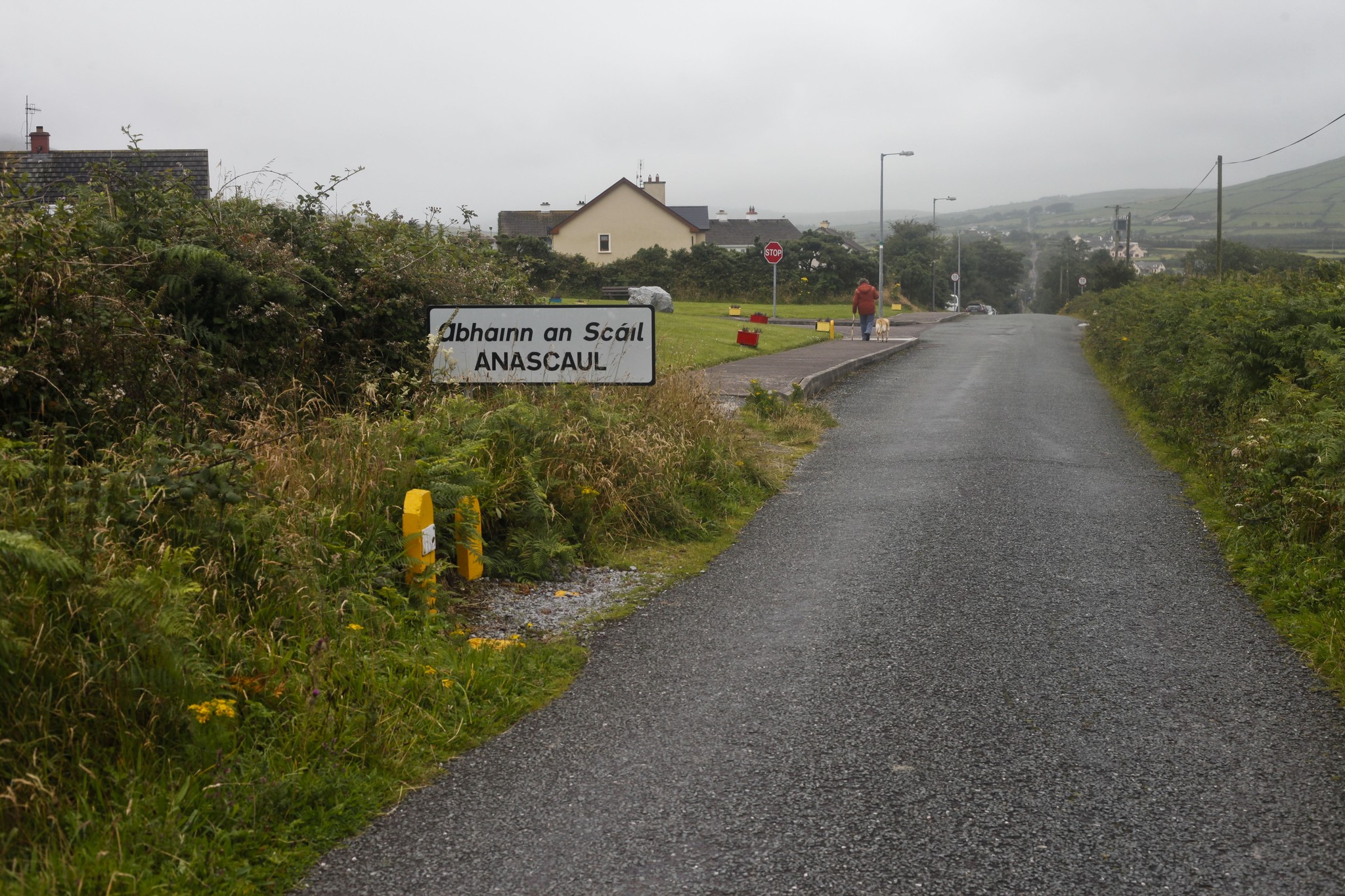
750;314;768;324
814;320;830;332
549;297;562;303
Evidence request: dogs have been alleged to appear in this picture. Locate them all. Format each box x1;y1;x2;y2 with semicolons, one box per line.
875;318;890;342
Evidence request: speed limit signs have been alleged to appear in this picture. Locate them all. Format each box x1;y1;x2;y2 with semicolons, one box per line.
951;272;960;282
1078;276;1087;286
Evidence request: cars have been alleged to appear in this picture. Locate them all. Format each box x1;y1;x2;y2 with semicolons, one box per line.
965;303;999;315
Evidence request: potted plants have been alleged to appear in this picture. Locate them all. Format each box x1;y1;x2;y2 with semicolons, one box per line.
729;302;741;316
736;324;764;345
573;299;588;304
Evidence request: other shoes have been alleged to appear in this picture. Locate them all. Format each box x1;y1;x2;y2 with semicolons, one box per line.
865;333;870;341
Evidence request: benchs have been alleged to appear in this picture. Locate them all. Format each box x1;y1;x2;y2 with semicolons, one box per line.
601;286;636;300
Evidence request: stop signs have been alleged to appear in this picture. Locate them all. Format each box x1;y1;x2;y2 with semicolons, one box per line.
764;242;783;264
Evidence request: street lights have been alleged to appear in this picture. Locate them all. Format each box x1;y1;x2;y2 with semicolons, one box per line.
878;151;914;319
931;197;957;313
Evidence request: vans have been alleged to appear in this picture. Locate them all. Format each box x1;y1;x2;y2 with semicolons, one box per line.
944;294;958;312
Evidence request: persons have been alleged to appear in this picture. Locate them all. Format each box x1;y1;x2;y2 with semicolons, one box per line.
853;278;879;341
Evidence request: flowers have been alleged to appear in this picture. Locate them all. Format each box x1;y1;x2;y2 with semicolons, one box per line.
548;291;561;298
817;317;830;322
754;312;766;316
